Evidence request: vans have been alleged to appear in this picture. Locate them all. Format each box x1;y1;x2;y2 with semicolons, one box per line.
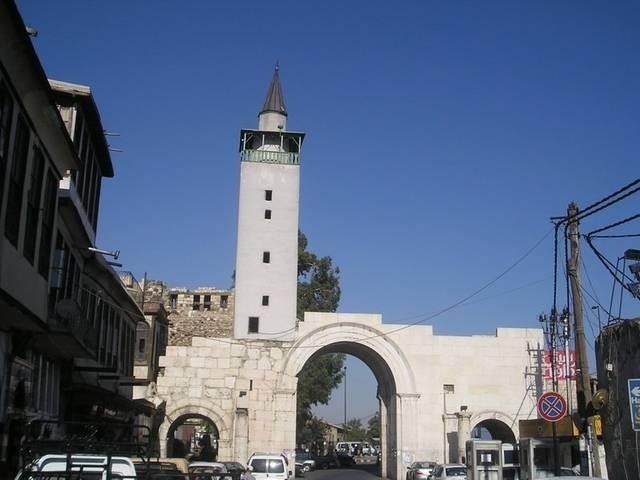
248;452;292;480
335;441;375;456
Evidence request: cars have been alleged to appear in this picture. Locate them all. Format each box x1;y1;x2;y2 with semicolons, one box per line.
406;461;468;480
295;452;355;472
14;453;256;480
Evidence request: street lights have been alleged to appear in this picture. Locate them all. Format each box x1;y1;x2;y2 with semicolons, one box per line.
592;305;601;331
